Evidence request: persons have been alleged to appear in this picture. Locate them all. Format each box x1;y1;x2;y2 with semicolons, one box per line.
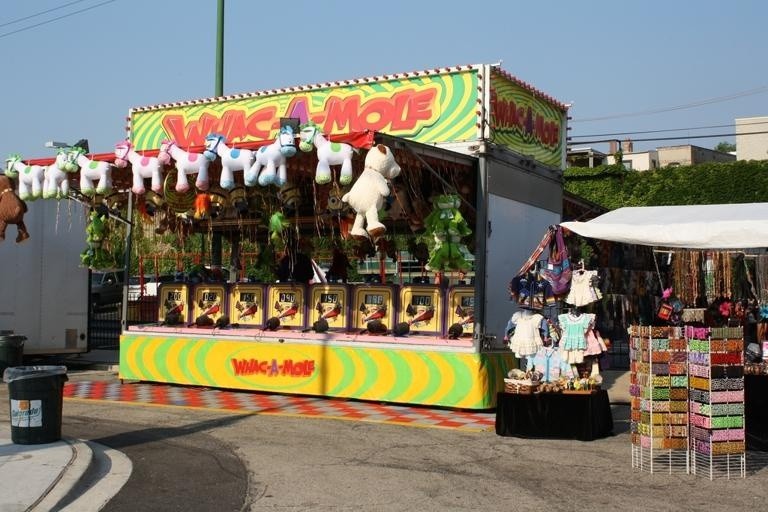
289;238;315;283
331;246;354;284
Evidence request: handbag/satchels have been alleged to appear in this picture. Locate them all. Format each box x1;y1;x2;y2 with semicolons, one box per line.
540;227;572;295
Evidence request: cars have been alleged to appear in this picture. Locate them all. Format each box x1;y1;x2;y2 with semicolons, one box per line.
125;273;175;303
91;268;124;313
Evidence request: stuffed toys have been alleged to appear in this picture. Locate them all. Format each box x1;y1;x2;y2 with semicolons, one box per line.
246;125;298;187
113;140;164;194
41;148;78;200
159;140;216;193
145;186;347;220
0;177;29;243
204;134;258;189
299;122;360;186
415;192;473;272
76;189;130;274
268;211;292;252
4;154;47;202
342;142;402;241
66;146;116;198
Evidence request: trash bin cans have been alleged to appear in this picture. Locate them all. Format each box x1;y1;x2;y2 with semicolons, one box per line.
0;330;27;378
6;365;67;444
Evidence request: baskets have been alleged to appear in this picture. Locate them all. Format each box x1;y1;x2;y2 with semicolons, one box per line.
504;378;540;394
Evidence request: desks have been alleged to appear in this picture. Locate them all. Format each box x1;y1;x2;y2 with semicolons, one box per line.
495;381;613;441
